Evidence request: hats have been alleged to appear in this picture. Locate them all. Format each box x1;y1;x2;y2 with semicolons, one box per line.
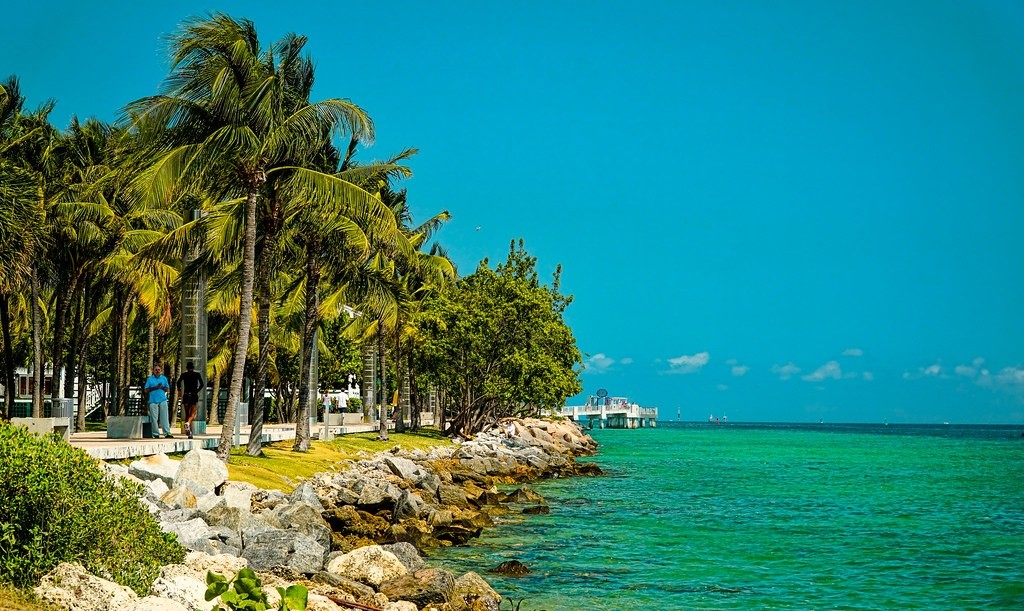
186;362;194;369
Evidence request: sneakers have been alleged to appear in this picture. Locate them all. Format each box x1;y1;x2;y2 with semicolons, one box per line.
183;421;191;436
188;432;193;439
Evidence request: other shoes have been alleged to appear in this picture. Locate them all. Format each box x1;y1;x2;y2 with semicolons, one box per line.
165;434;173;438
154;434;159;438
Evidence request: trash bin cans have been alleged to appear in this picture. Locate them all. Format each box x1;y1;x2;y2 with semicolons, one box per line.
51;397;74;434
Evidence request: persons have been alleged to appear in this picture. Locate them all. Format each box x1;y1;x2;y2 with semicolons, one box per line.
144;364;174;439
391;388;398;422
504;421;515;439
177;362;203;439
321;390;332;413
335;388;350;413
